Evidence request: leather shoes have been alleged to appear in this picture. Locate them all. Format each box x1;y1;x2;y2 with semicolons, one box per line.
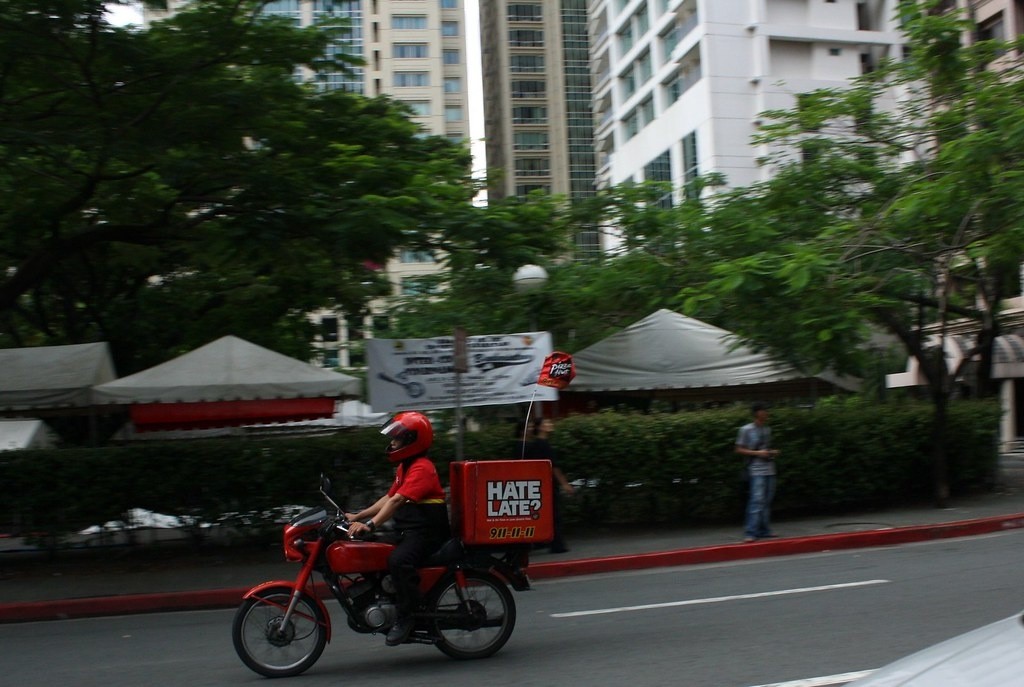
385;615;416;646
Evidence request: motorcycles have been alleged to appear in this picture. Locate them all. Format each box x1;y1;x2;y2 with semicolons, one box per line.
231;489;535;678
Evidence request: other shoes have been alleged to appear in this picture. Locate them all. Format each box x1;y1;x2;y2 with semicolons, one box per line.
743;536;760;542
761;533;778;537
551;549;568;553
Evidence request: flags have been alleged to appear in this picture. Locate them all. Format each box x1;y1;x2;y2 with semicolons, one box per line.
538;352;577;389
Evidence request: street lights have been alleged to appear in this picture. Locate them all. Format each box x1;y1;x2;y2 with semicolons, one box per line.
512;263;551;435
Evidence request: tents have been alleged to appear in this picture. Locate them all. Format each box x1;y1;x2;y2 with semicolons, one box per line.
0;309;868;454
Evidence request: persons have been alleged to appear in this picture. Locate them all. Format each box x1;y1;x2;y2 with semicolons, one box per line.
335;410;448;646
511;418;570;555
737;403;781;542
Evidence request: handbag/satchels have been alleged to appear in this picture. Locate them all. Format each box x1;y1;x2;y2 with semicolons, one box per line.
738;467;750;491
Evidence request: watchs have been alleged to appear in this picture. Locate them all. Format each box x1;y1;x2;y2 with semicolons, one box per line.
365;519;376;533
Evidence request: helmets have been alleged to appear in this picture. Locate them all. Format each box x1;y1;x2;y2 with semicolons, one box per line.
378;412;432;463
